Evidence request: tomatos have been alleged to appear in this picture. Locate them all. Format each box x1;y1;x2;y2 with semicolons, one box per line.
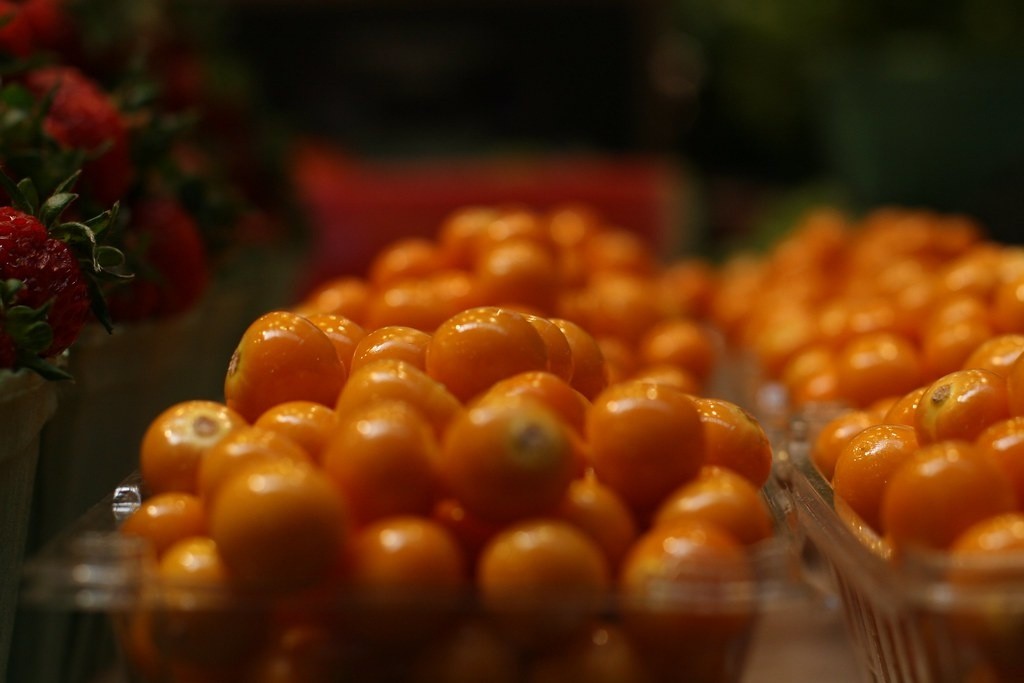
116;199;1024;683
